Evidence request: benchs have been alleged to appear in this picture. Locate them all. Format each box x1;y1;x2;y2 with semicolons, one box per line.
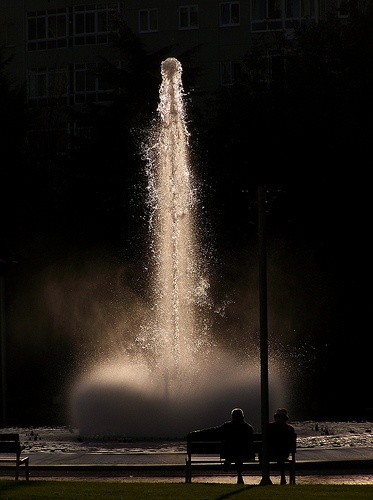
184;430;297;485
0;432;29;482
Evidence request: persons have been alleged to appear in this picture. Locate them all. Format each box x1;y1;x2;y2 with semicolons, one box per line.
189;408;257;484
267;407;296;485
